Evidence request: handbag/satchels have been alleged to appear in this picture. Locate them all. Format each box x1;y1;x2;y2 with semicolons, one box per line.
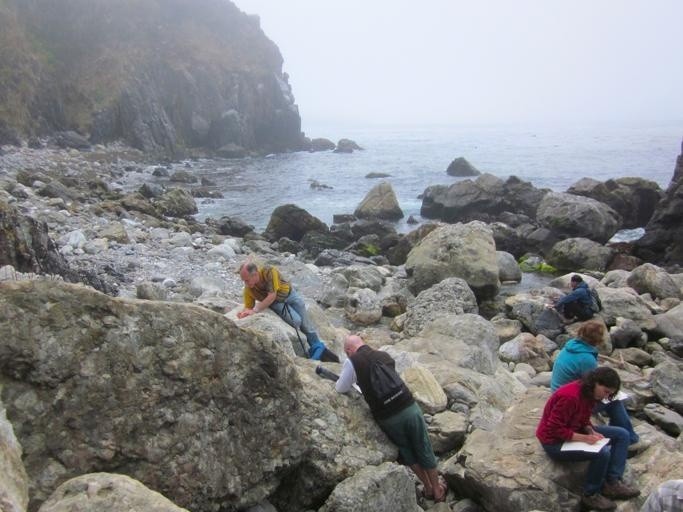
590;287;601;313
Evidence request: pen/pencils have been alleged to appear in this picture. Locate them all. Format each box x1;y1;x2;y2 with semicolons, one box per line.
583;425;595;435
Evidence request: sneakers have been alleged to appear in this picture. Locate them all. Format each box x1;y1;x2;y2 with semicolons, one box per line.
603;480;640;499
582;493;617;510
627;438;651;451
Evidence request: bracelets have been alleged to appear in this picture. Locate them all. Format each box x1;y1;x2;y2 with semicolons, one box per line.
253;307;258;313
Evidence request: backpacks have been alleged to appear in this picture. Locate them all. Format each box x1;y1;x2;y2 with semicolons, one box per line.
370;360;404;407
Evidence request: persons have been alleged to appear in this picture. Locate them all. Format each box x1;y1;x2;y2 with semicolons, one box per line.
548;318;653;461
235;260;319;347
535;367;642;511
334;334;449;505
545;275;595;324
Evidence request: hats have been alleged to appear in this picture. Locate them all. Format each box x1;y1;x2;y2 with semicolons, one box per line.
571;275;582;282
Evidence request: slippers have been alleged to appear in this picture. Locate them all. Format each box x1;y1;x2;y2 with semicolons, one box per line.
423;482;449;502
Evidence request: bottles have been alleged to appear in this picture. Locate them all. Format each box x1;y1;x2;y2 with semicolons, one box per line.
316;365;339;381
